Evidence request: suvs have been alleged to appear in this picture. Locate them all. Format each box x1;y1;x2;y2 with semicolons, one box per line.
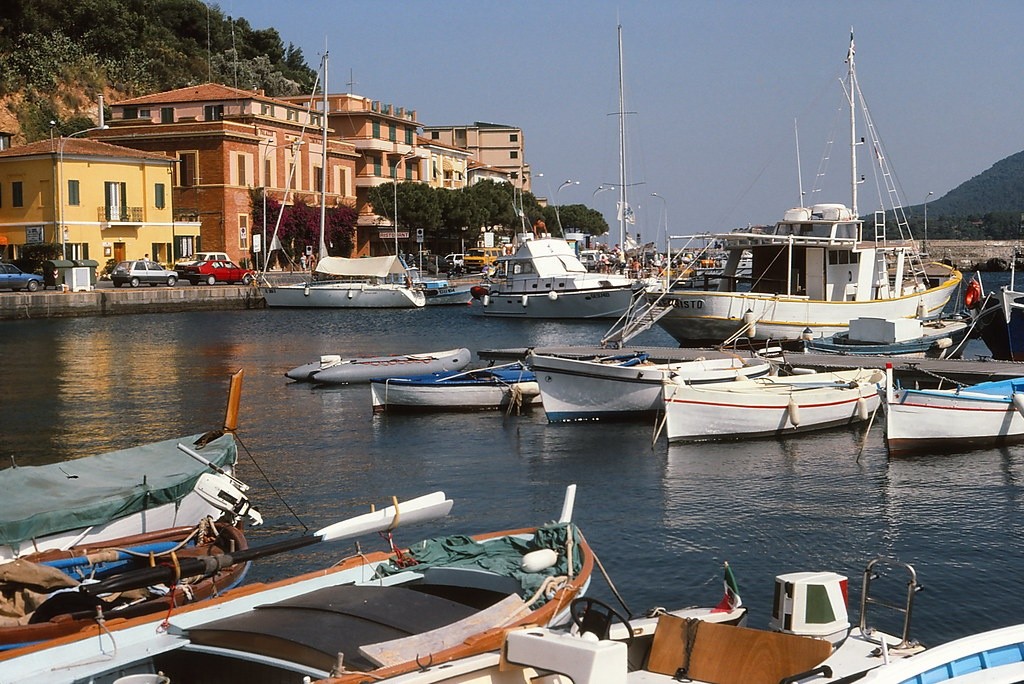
174;252;232;279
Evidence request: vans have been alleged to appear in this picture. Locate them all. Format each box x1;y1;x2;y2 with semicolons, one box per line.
464;247;503;273
443;253;464;269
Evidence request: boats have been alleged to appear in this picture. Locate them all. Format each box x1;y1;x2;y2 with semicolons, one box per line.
0;485;595;684
283;346;1024;453
465;188;664;319
0;369;255;651
370;558;1024;684
601;25;1024;358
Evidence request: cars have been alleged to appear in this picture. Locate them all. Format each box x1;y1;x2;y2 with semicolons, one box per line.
0;264;45;292
110;260;179;287
182;260;256;286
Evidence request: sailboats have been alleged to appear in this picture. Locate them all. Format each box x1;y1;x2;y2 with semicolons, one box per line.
257;33;474;308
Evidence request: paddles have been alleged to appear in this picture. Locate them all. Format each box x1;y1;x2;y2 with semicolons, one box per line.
79;491;454;597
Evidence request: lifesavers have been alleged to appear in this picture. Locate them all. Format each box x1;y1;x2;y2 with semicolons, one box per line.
964;279;981;310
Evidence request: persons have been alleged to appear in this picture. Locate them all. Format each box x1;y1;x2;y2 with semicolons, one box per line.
532;217;548;240
143;254;150;269
579;242;585;251
454;264;460;272
588;231;665;278
300;253;315;270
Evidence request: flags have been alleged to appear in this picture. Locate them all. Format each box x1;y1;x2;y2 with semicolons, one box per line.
617;201;636;224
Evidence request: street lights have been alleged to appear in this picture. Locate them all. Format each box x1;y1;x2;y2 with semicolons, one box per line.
512;163;545;205
460;161;493;192
924;192;934;248
262;138;306;272
393;150;427;256
591;186;614;207
650;192;668;251
59;125;110;260
555;180;581;221
